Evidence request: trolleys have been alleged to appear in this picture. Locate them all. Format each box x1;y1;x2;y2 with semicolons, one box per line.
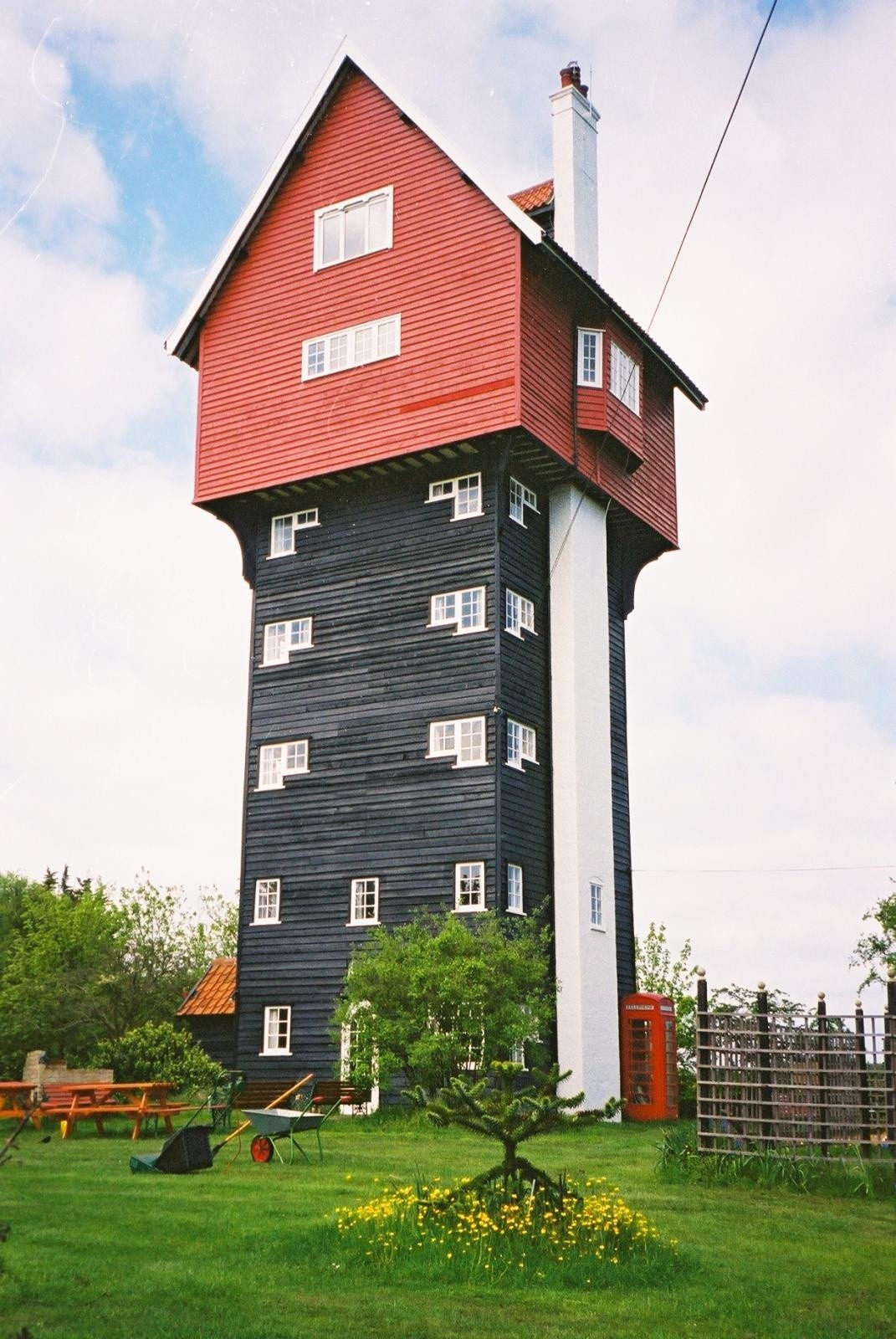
240;1095;353;1166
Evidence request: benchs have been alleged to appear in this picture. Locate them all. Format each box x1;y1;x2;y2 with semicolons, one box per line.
47;1103;229;1113
44;1083;117;1108
311;1081;371;1118
234;1081;297;1105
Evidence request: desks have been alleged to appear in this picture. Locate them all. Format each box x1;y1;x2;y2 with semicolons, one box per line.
62;1082;175;1139
0;1082;40;1131
